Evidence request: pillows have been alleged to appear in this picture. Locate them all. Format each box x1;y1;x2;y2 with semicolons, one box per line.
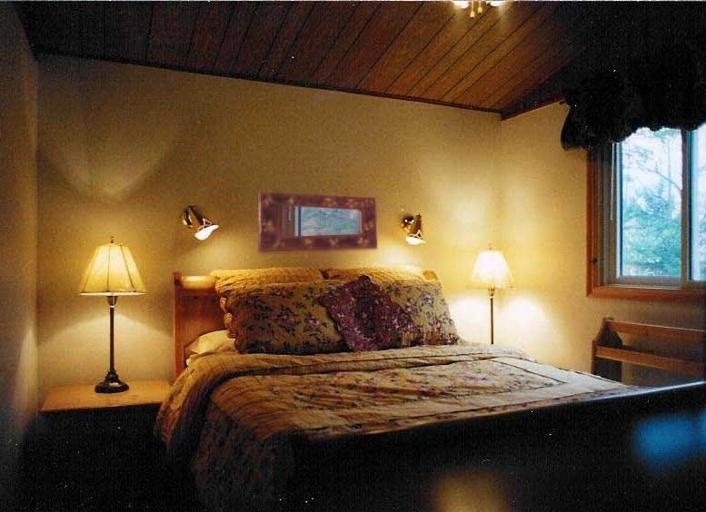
214;268;460;354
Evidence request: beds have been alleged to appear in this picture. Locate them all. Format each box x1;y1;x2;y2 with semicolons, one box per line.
171;267;706;509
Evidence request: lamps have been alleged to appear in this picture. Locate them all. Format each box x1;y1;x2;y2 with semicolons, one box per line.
467;245;517;345
75;233;147;393
179;203;220;242
400;213;427;247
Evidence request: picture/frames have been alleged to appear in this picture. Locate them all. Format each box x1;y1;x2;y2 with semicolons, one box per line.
256;190;379;251
